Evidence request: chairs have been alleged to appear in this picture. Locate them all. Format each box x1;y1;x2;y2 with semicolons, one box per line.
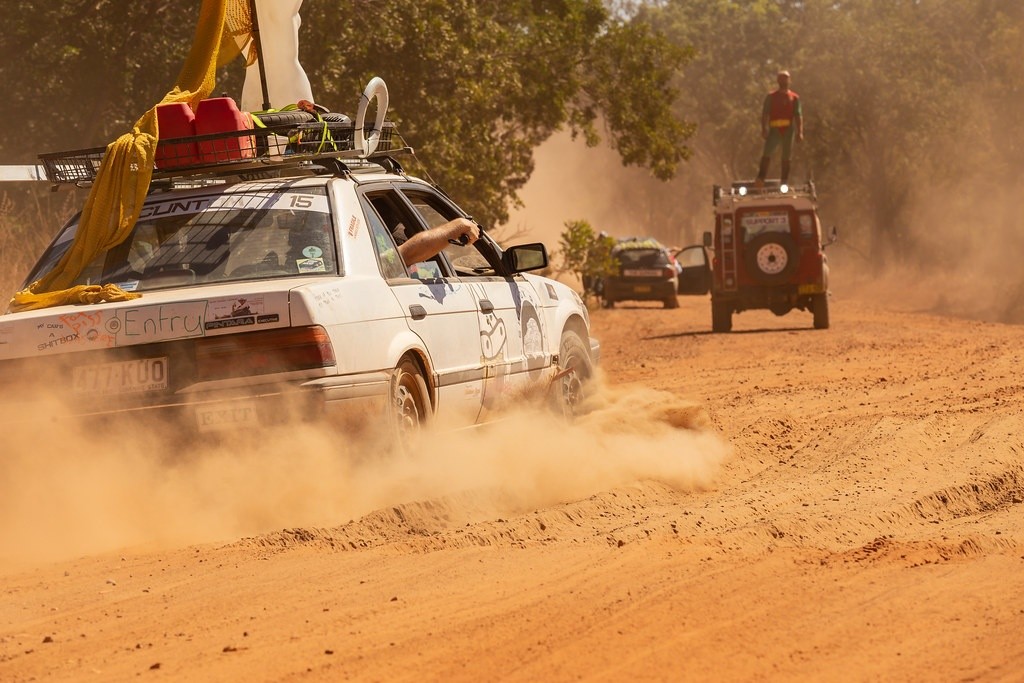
142;253;211;283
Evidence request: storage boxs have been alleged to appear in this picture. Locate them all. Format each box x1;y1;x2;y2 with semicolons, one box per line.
145;97;194;165
193;92;255;162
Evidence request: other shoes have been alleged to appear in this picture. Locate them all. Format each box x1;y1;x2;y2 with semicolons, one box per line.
750;179;764;193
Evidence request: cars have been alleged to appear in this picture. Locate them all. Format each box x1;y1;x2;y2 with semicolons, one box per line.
583;235;711;311
1;121;603;469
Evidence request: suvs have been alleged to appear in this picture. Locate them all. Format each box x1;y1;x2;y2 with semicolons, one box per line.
702;179;837;332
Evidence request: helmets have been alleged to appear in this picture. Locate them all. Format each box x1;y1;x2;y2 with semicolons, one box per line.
778;71;790;80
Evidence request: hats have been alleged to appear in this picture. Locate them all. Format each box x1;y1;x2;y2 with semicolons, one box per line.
369;194;403;232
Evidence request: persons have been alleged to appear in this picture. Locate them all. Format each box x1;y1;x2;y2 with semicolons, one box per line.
756;70;804;186
288;212;480;277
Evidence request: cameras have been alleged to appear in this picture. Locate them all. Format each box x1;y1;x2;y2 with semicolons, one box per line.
458;215;485;245
